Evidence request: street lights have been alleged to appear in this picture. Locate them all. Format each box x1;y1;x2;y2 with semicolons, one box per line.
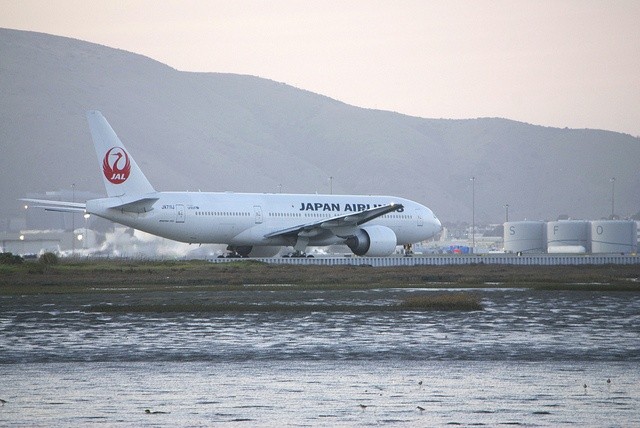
469;177;478;247
71;182;76;256
327;175;334;196
503;202;510;222
82;213;89;257
607;177;615;218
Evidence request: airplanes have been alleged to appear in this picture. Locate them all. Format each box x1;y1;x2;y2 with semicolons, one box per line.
16;111;442;257
